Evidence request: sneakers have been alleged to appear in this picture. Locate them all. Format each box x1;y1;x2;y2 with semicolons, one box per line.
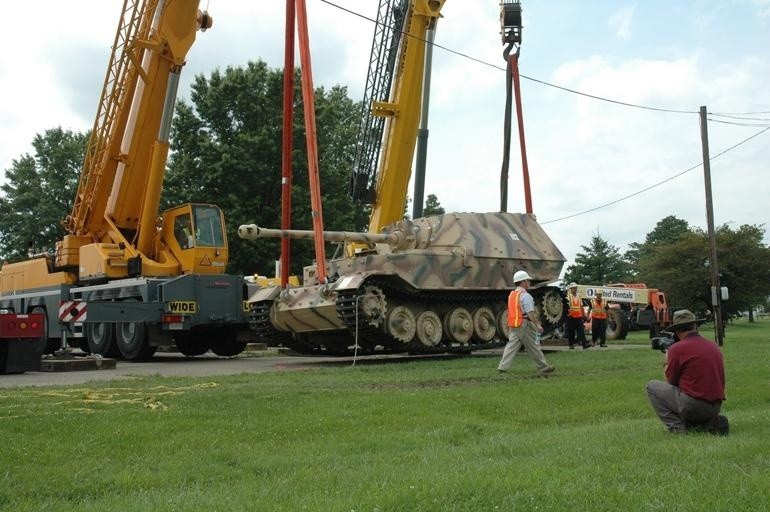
715;415;729;436
569;340;608;349
538;365;555;374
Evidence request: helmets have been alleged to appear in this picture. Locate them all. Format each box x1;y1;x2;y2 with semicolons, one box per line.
513;270;534;283
595;288;603;295
569;282;578;288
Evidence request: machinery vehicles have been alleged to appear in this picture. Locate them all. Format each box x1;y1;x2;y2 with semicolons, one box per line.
236;212;569;357
554;282;670;340
0;0;252;361
243;0;448;281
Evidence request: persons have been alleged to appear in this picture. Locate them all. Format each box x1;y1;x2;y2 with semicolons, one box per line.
561;282;592;350
645;309;730;439
587;287;615;348
179;217;204;249
497;269;556;374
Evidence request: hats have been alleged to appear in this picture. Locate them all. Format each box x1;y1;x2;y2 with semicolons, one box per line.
665;309;706;330
